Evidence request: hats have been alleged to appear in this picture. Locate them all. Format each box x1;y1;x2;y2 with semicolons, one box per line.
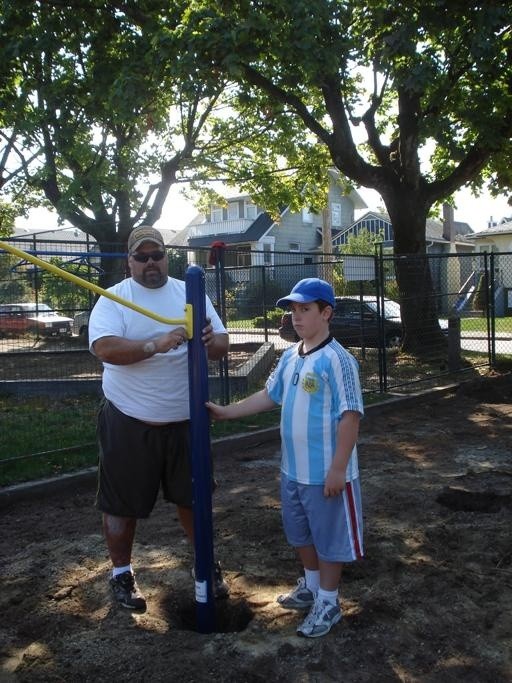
275;276;337;308
127;225;165;254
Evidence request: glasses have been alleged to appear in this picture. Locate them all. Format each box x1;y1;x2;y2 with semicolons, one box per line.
130;248;167;262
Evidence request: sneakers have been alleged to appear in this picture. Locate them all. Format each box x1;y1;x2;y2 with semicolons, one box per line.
107;571;147;612
190;560;232;600
296;598;343;639
275;578;317;608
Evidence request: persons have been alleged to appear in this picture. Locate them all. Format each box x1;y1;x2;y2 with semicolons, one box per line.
205;277;365;639
88;225;230;610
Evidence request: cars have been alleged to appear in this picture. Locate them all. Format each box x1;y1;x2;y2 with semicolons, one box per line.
71;307;91;336
0;299;74;337
276;292;460;352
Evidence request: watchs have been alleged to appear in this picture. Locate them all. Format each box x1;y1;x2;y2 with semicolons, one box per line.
143;342;155;353
